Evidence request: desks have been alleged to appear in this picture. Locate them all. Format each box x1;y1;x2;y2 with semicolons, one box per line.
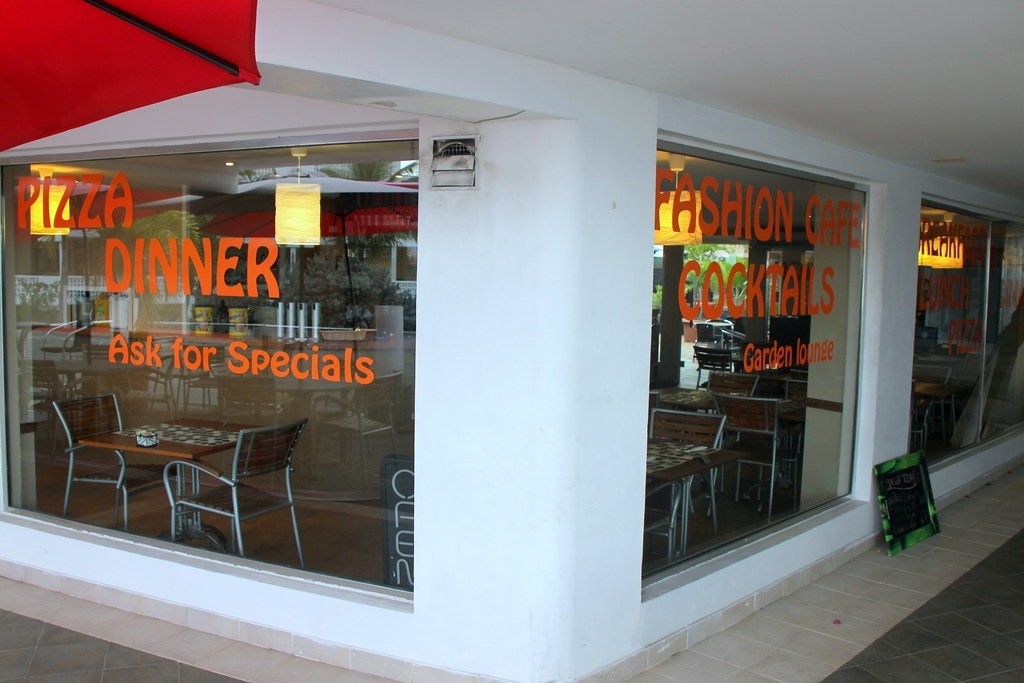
52;360;147;396
643;439;725;497
913;379;962;448
78;417;267;554
651;387;806;506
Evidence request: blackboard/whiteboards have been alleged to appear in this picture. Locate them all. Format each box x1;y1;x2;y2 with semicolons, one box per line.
873;450;941;557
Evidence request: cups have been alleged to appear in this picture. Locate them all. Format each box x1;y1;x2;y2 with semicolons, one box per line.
229;308;248;337
194;306;213;334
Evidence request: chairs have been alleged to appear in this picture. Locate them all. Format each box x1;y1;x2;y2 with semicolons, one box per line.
18;359;76;455
914;326;940;353
162;416;310;570
126;359;180;422
52;392;179;532
216;376;288;425
209;363;291;425
914;365;954;453
708;371;759;502
707;392;795;523
693;344;734;389
648;410;729;562
757;379;808;502
183;345;226;416
314;369;404;488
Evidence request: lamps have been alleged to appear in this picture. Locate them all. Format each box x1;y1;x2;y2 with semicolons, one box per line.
653;152;704;246
274;147;322;243
30;168;70;235
918;222;964;269
